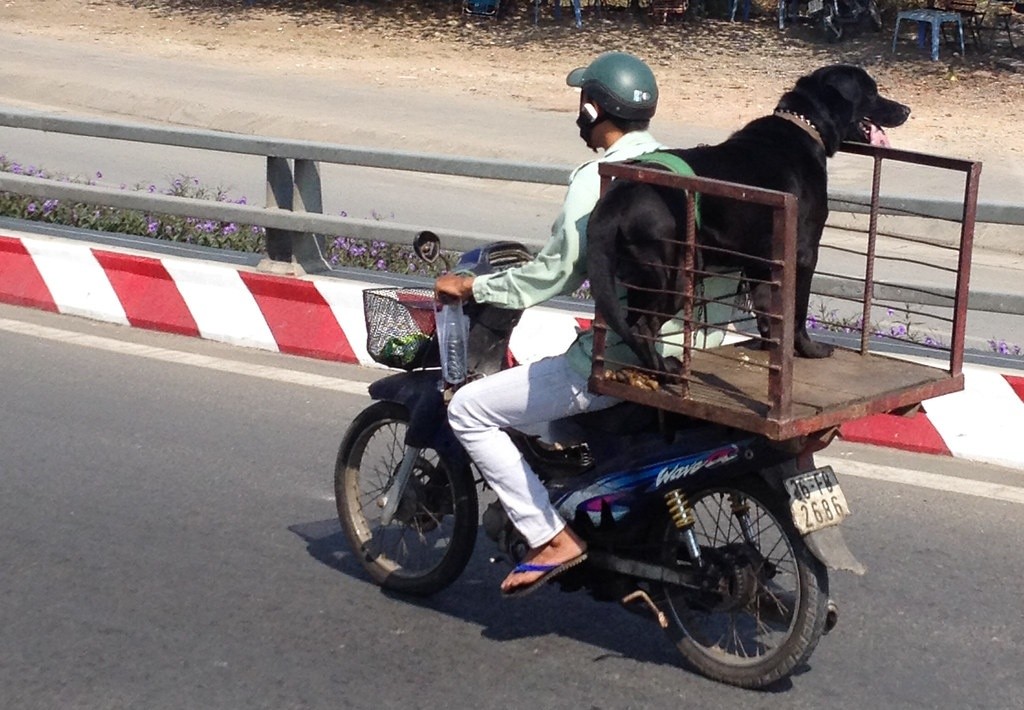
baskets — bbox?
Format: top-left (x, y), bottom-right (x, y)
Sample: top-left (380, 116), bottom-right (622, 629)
top-left (361, 286), bottom-right (441, 368)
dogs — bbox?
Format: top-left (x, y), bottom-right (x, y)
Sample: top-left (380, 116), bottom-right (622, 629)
top-left (585, 65), bottom-right (911, 384)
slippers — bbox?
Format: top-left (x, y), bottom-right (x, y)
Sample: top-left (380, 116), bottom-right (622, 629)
top-left (500, 550), bottom-right (587, 600)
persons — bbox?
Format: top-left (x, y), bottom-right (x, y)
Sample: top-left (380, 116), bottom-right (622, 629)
top-left (431, 51), bottom-right (742, 601)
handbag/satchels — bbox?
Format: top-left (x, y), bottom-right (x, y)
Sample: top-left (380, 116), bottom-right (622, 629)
top-left (433, 295), bottom-right (469, 384)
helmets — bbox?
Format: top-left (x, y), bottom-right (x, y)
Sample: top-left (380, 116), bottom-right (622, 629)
top-left (566, 52), bottom-right (658, 120)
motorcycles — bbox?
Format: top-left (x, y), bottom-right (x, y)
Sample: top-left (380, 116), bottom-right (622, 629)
top-left (329, 226), bottom-right (875, 686)
